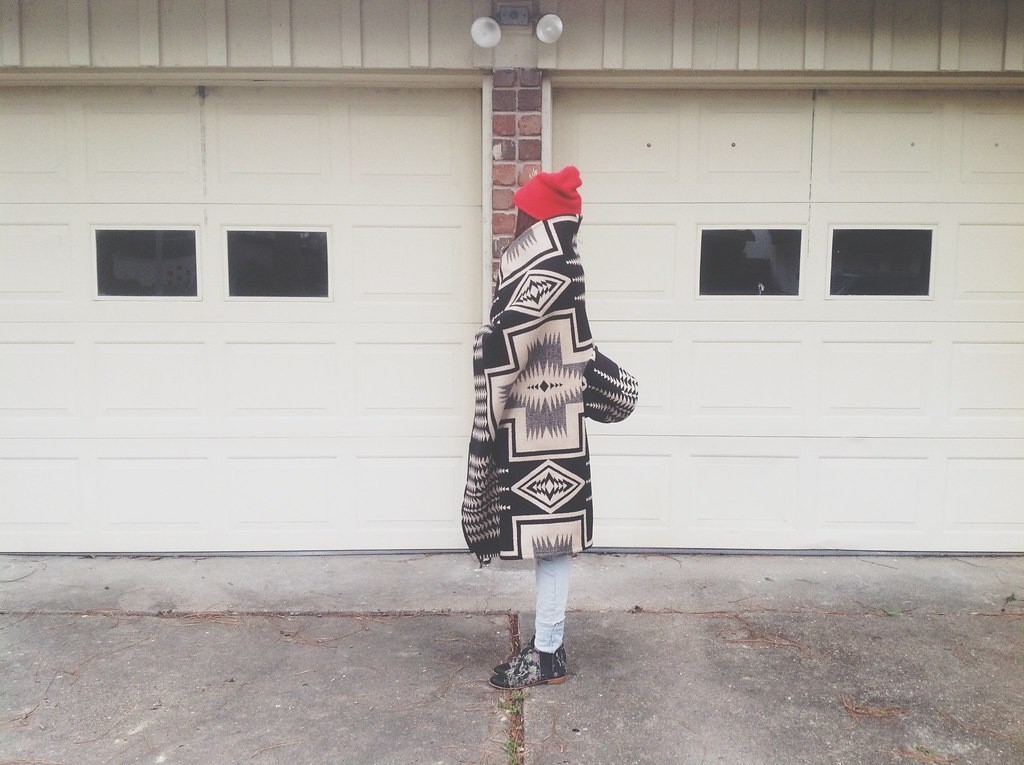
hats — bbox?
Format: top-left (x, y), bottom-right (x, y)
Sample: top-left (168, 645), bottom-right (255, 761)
top-left (512, 165), bottom-right (583, 220)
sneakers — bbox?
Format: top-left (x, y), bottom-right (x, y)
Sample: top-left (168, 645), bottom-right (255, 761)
top-left (493, 635), bottom-right (535, 672)
top-left (489, 645), bottom-right (566, 689)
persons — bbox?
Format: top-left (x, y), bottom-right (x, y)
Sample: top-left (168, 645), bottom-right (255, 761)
top-left (460, 165), bottom-right (640, 692)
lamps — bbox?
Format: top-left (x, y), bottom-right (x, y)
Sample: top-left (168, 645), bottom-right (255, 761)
top-left (531, 10), bottom-right (564, 45)
top-left (470, 13), bottom-right (506, 49)
